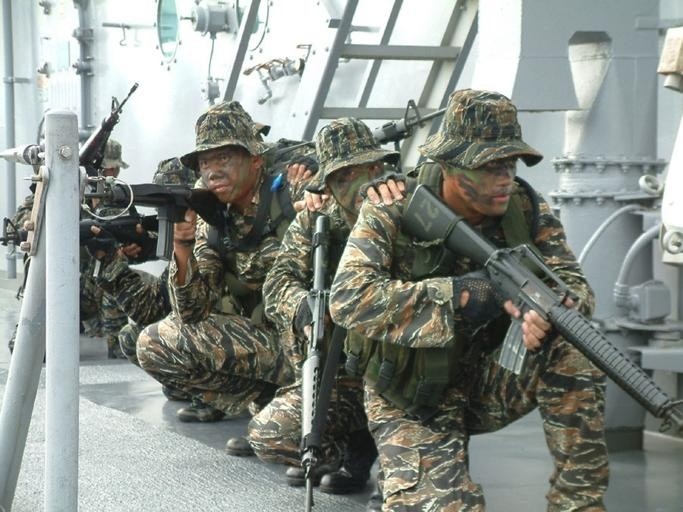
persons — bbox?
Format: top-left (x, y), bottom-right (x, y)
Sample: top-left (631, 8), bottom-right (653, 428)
top-left (328, 87), bottom-right (617, 511)
top-left (132, 100), bottom-right (318, 457)
top-left (248, 118), bottom-right (413, 495)
top-left (84, 153), bottom-right (204, 402)
top-left (4, 136), bottom-right (135, 363)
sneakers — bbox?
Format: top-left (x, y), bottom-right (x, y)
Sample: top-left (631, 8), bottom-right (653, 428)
top-left (286, 460), bottom-right (366, 493)
top-left (175, 396), bottom-right (227, 421)
top-left (226, 435), bottom-right (254, 456)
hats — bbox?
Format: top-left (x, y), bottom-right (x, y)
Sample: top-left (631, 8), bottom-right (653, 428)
top-left (304, 116), bottom-right (401, 193)
top-left (99, 138), bottom-right (129, 170)
top-left (418, 89), bottom-right (544, 169)
top-left (180, 100), bottom-right (277, 171)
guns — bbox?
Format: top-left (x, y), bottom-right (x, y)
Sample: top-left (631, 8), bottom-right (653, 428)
top-left (268, 100), bottom-right (447, 190)
top-left (83, 183), bottom-right (227, 259)
top-left (79, 82), bottom-right (138, 174)
top-left (0, 215), bottom-right (159, 246)
top-left (403, 184), bottom-right (683, 437)
top-left (296, 215), bottom-right (330, 512)
